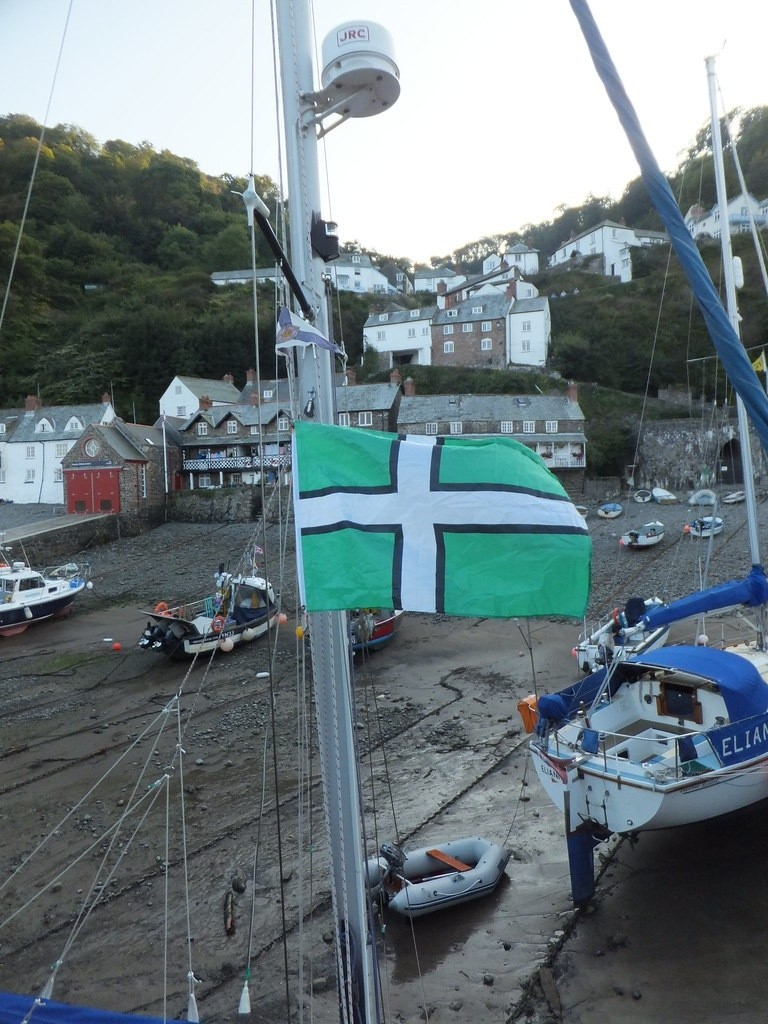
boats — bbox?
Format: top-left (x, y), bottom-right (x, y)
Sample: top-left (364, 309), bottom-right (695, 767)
top-left (596, 503), bottom-right (623, 520)
top-left (515, 56), bottom-right (768, 839)
top-left (688, 488), bottom-right (716, 507)
top-left (575, 505), bottom-right (589, 520)
top-left (356, 839), bottom-right (514, 920)
top-left (651, 486), bottom-right (679, 504)
top-left (293, 607), bottom-right (408, 657)
top-left (0, 551), bottom-right (92, 637)
top-left (722, 490), bottom-right (746, 504)
top-left (139, 572), bottom-right (285, 659)
top-left (619, 520), bottom-right (664, 551)
top-left (687, 516), bottom-right (724, 537)
top-left (634, 490), bottom-right (651, 504)
top-left (567, 595), bottom-right (676, 674)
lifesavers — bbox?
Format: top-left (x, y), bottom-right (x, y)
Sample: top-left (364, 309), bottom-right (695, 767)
top-left (209, 615), bottom-right (225, 633)
top-left (612, 607), bottom-right (623, 627)
top-left (153, 602), bottom-right (168, 614)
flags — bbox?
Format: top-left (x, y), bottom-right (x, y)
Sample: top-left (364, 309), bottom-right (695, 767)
top-left (254, 544), bottom-right (263, 555)
top-left (289, 418), bottom-right (595, 619)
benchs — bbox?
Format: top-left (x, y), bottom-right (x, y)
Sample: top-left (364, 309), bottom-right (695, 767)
top-left (598, 720), bottom-right (697, 764)
top-left (427, 850), bottom-right (472, 872)
